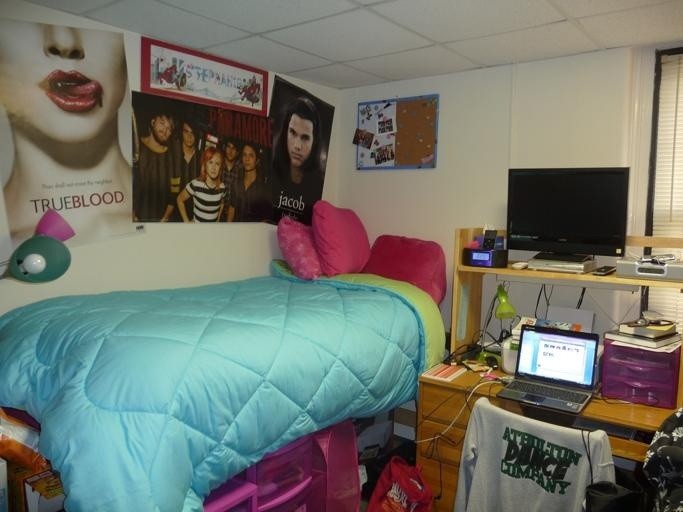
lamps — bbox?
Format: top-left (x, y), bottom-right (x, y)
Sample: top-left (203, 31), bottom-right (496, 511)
top-left (476, 284), bottom-right (516, 364)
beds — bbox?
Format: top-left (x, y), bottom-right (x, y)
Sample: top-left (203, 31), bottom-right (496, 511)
top-left (0, 260), bottom-right (446, 512)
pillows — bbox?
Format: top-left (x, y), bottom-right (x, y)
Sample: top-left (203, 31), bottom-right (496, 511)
top-left (312, 200), bottom-right (371, 277)
top-left (360, 235), bottom-right (447, 307)
top-left (278, 216), bottom-right (322, 280)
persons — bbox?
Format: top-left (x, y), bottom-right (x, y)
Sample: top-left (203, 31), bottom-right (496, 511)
top-left (219, 138), bottom-right (241, 221)
top-left (175, 147), bottom-right (227, 223)
top-left (0, 18), bottom-right (139, 267)
top-left (225, 141), bottom-right (267, 222)
top-left (131, 109), bottom-right (181, 222)
top-left (273, 99), bottom-right (323, 227)
top-left (174, 117), bottom-right (202, 224)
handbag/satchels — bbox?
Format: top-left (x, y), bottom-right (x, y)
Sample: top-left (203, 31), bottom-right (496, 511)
top-left (366, 455), bottom-right (436, 512)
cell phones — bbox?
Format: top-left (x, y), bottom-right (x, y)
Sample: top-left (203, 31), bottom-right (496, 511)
top-left (592, 266), bottom-right (616, 276)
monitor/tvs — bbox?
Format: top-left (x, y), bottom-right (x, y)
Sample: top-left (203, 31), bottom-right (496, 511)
top-left (508, 167), bottom-right (629, 263)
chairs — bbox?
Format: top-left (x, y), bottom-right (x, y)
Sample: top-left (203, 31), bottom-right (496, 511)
top-left (453, 397), bottom-right (616, 512)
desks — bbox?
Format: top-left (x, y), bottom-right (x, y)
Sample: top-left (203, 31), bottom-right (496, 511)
top-left (416, 227), bottom-right (683, 512)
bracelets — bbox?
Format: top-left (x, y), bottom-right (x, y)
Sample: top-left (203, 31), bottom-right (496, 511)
top-left (160, 217), bottom-right (167, 222)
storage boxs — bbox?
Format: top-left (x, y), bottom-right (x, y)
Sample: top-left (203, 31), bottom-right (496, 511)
top-left (601, 339), bottom-right (680, 410)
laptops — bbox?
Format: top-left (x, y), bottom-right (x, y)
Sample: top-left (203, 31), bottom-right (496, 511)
top-left (500, 326), bottom-right (600, 415)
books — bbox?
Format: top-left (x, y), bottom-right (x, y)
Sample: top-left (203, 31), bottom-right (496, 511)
top-left (603, 329), bottom-right (680, 349)
top-left (509, 315), bottom-right (582, 350)
top-left (618, 319), bottom-right (677, 339)
